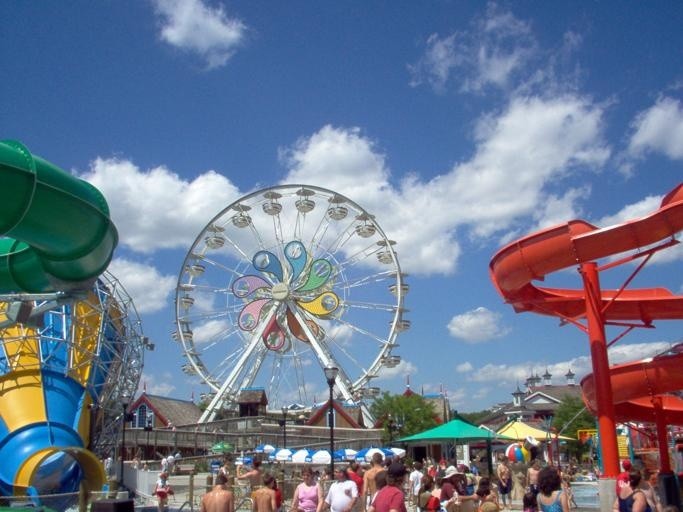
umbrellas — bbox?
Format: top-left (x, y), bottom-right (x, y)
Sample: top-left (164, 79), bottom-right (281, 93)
top-left (393, 418), bottom-right (512, 465)
top-left (495, 418), bottom-right (578, 443)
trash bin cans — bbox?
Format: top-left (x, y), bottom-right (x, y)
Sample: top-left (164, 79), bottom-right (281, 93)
top-left (104, 458), bottom-right (113, 479)
top-left (211, 461), bottom-right (222, 473)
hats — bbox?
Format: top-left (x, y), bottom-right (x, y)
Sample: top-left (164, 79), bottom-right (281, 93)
top-left (442, 466), bottom-right (464, 479)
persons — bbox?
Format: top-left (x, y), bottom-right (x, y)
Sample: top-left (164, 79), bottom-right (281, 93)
top-left (105, 448), bottom-right (682, 512)
